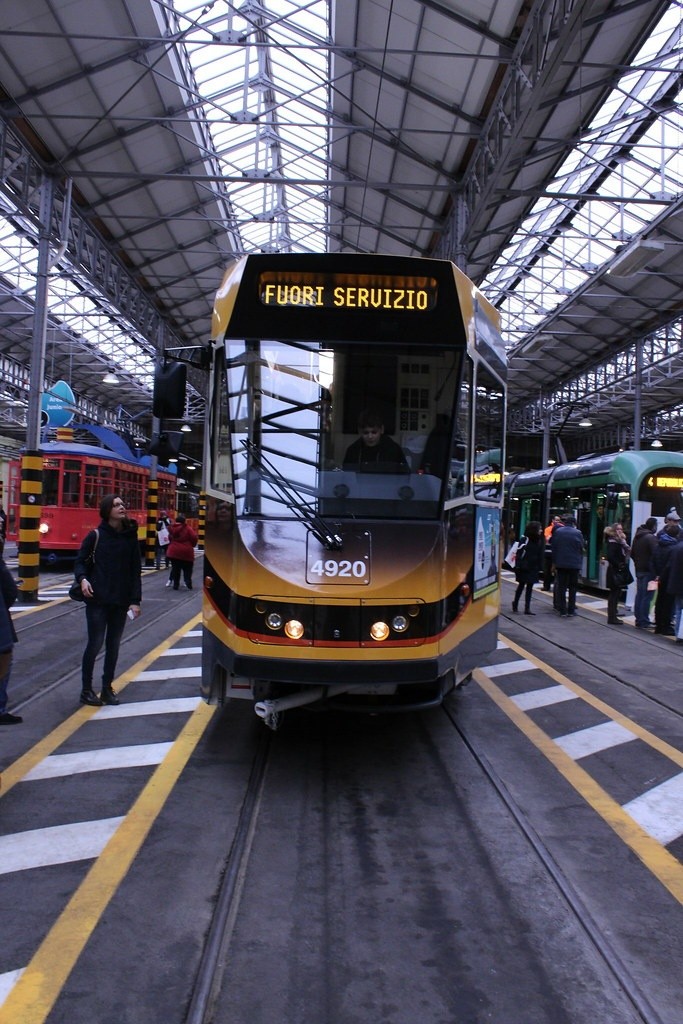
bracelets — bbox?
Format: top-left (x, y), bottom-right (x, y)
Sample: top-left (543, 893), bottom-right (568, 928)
top-left (80, 579), bottom-right (86, 582)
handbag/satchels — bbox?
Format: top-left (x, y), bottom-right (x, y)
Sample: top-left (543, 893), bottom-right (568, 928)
top-left (505, 542), bottom-right (526, 569)
top-left (157, 522), bottom-right (171, 546)
top-left (69, 580), bottom-right (87, 603)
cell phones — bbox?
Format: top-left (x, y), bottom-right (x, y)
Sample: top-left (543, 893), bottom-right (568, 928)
top-left (127, 609), bottom-right (134, 620)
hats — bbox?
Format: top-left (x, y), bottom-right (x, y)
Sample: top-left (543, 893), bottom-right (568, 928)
top-left (668, 513), bottom-right (681, 520)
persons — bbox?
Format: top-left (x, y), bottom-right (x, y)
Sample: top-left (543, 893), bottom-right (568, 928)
top-left (605, 523), bottom-right (634, 625)
top-left (0, 506), bottom-right (23, 725)
top-left (155, 510), bottom-right (172, 569)
top-left (630, 518), bottom-right (658, 628)
top-left (73, 494), bottom-right (142, 706)
top-left (654, 512), bottom-right (683, 646)
top-left (542, 513), bottom-right (579, 609)
top-left (342, 415), bottom-right (410, 474)
top-left (552, 517), bottom-right (584, 617)
top-left (163, 515), bottom-right (198, 590)
top-left (511, 521), bottom-right (543, 616)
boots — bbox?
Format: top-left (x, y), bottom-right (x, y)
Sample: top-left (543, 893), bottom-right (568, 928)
top-left (525, 604), bottom-right (536, 616)
top-left (511, 601), bottom-right (519, 613)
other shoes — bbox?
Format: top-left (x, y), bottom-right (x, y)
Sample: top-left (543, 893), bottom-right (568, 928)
top-left (663, 629), bottom-right (675, 635)
top-left (155, 567), bottom-right (161, 571)
top-left (174, 586), bottom-right (179, 590)
top-left (654, 627), bottom-right (662, 634)
top-left (643, 622), bottom-right (657, 629)
top-left (560, 612), bottom-right (569, 616)
top-left (568, 612), bottom-right (577, 616)
top-left (635, 623), bottom-right (642, 628)
top-left (188, 585), bottom-right (193, 590)
top-left (541, 586), bottom-right (553, 592)
top-left (1, 713), bottom-right (25, 726)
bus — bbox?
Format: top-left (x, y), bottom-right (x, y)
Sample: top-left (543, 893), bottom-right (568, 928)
top-left (5, 426), bottom-right (198, 573)
top-left (153, 253), bottom-right (507, 719)
top-left (502, 447), bottom-right (683, 590)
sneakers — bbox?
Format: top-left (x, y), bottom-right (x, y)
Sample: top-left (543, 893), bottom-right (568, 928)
top-left (166, 579), bottom-right (173, 586)
top-left (81, 691), bottom-right (104, 704)
top-left (609, 618), bottom-right (624, 625)
top-left (100, 686), bottom-right (120, 705)
